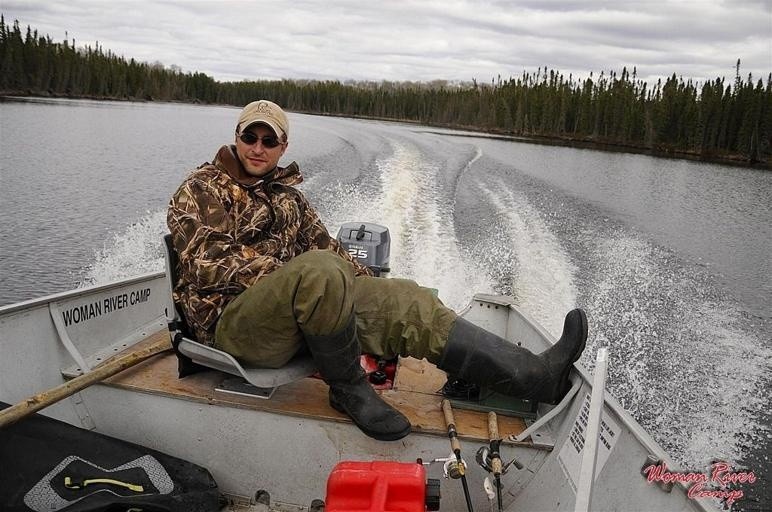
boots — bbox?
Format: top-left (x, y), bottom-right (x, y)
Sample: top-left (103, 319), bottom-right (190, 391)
top-left (435, 309), bottom-right (589, 404)
top-left (300, 305), bottom-right (411, 442)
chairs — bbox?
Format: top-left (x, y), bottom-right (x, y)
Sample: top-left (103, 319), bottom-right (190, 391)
top-left (161, 233), bottom-right (319, 388)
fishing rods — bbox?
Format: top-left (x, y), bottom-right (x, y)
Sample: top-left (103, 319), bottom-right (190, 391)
top-left (417, 399), bottom-right (474, 511)
top-left (475, 410), bottom-right (523, 511)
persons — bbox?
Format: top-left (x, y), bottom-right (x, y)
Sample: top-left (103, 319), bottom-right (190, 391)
top-left (164, 99), bottom-right (588, 441)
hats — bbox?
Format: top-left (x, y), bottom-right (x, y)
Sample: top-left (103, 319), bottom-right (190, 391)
top-left (238, 99), bottom-right (288, 138)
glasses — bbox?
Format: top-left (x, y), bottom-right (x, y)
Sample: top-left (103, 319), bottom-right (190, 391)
top-left (239, 128), bottom-right (281, 148)
